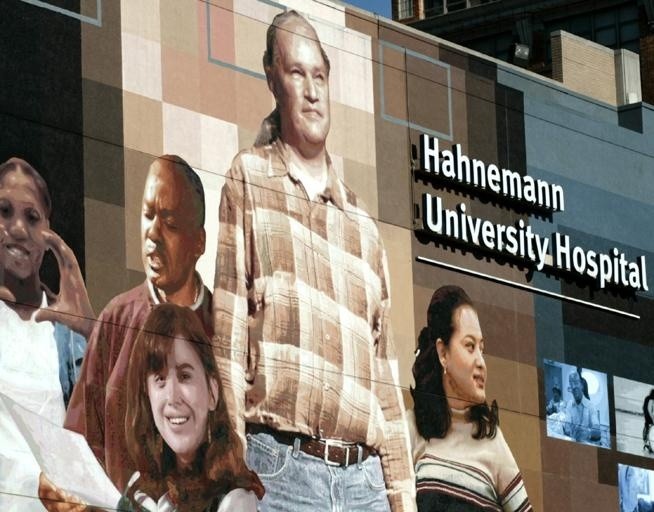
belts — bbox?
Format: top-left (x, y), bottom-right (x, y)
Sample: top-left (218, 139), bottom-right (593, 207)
top-left (248, 424), bottom-right (377, 467)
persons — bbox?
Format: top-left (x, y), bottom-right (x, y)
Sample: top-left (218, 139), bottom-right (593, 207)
top-left (619, 463), bottom-right (654, 512)
top-left (108, 303), bottom-right (269, 511)
top-left (207, 9), bottom-right (420, 512)
top-left (642, 388), bottom-right (654, 454)
top-left (404, 283), bottom-right (533, 509)
top-left (545, 384), bottom-right (565, 414)
top-left (0, 155), bottom-right (94, 509)
top-left (563, 372), bottom-right (601, 442)
top-left (34, 152), bottom-right (212, 509)
top-left (576, 366), bottom-right (589, 398)
